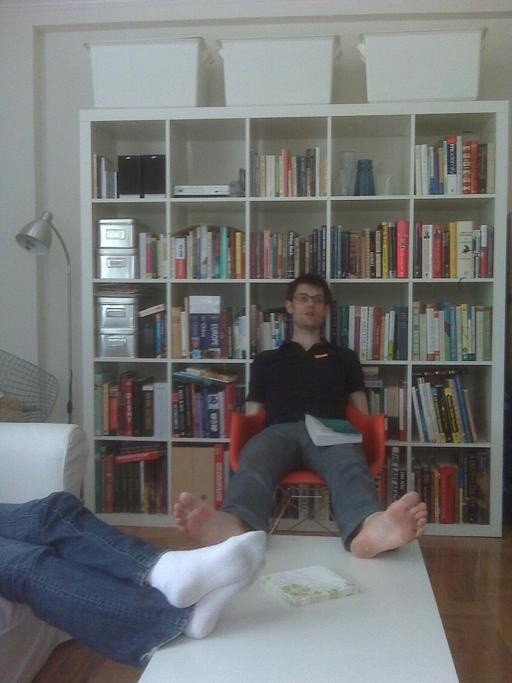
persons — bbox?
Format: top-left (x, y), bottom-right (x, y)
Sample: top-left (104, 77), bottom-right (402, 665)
top-left (171, 272), bottom-right (430, 559)
top-left (0, 491), bottom-right (268, 670)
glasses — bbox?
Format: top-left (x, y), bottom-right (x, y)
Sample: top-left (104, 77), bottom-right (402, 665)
top-left (293, 293), bottom-right (325, 304)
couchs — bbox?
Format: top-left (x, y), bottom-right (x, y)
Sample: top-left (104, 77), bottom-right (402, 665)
top-left (1, 417), bottom-right (86, 682)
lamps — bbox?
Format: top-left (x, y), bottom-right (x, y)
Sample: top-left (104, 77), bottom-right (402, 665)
top-left (13, 210), bottom-right (76, 423)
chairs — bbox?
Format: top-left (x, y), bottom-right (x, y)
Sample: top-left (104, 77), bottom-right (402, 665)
top-left (227, 402), bottom-right (386, 534)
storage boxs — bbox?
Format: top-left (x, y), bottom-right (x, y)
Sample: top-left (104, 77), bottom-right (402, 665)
top-left (98, 326), bottom-right (141, 358)
top-left (96, 294), bottom-right (143, 329)
top-left (213, 33), bottom-right (341, 107)
top-left (96, 248), bottom-right (140, 279)
top-left (356, 24), bottom-right (490, 102)
top-left (81, 33), bottom-right (212, 108)
top-left (97, 217), bottom-right (141, 248)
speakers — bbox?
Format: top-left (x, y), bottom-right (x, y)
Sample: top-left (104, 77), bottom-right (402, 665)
top-left (117, 155), bottom-right (141, 199)
top-left (142, 154), bottom-right (166, 198)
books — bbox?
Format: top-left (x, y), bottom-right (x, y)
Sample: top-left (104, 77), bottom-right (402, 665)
top-left (250, 147), bottom-right (325, 196)
top-left (414, 135), bottom-right (495, 193)
top-left (330, 220), bottom-right (410, 278)
top-left (251, 305), bottom-right (293, 359)
top-left (139, 224), bottom-right (246, 279)
top-left (171, 295), bottom-right (246, 358)
top-left (413, 220), bottom-right (494, 525)
top-left (172, 366), bottom-right (244, 439)
top-left (258, 564), bottom-right (357, 607)
top-left (93, 153), bottom-right (117, 198)
top-left (331, 298), bottom-right (408, 361)
top-left (249, 224), bottom-right (327, 279)
top-left (95, 371), bottom-right (168, 436)
top-left (95, 441), bottom-right (168, 514)
top-left (360, 366), bottom-right (407, 442)
top-left (210, 444), bottom-right (407, 518)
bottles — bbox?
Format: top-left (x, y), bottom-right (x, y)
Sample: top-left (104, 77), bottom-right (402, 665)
top-left (335, 151), bottom-right (393, 197)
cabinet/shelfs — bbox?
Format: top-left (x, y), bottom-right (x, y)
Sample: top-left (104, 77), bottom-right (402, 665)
top-left (78, 96), bottom-right (512, 539)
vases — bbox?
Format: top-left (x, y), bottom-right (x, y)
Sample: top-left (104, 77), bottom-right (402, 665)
top-left (330, 149), bottom-right (357, 197)
top-left (352, 158), bottom-right (376, 195)
